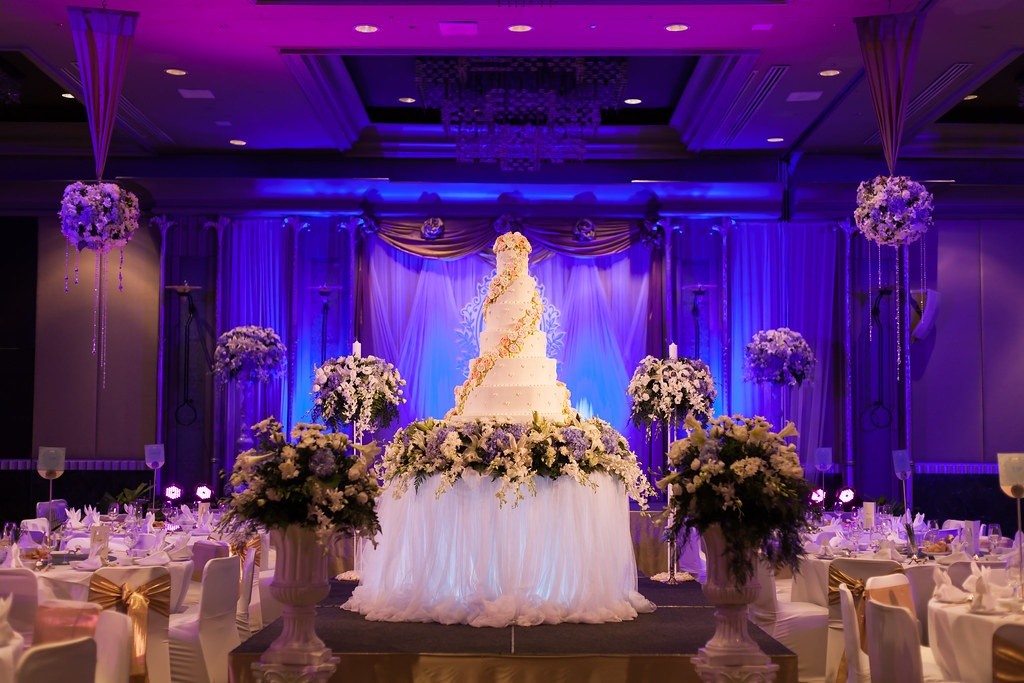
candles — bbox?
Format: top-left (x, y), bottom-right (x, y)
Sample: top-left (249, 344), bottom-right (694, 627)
top-left (352, 338), bottom-right (362, 359)
top-left (668, 342), bottom-right (678, 361)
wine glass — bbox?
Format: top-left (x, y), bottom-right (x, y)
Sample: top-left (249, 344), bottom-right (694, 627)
top-left (40, 496), bottom-right (258, 568)
top-left (773, 500), bottom-right (1024, 619)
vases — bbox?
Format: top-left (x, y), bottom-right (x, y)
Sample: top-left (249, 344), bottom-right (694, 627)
top-left (696, 522), bottom-right (773, 669)
top-left (259, 523), bottom-right (337, 666)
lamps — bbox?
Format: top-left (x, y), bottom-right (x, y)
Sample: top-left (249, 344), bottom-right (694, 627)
top-left (413, 55), bottom-right (629, 175)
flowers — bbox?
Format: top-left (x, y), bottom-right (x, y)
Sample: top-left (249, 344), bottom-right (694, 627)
top-left (742, 328), bottom-right (818, 390)
top-left (205, 325), bottom-right (289, 398)
top-left (645, 410), bottom-right (823, 599)
top-left (492, 214), bottom-right (524, 235)
top-left (421, 217), bottom-right (445, 240)
top-left (624, 355), bottom-right (718, 447)
top-left (492, 231), bottom-right (533, 255)
top-left (56, 181), bottom-right (142, 256)
top-left (444, 250), bottom-right (544, 420)
top-left (355, 213), bottom-right (381, 239)
top-left (572, 217), bottom-right (598, 242)
top-left (299, 352), bottom-right (408, 440)
top-left (639, 215), bottom-right (668, 252)
top-left (379, 414), bottom-right (660, 519)
top-left (853, 175), bottom-right (934, 249)
top-left (208, 413), bottom-right (383, 556)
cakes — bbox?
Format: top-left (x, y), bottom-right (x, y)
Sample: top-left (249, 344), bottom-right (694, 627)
top-left (444, 231), bottom-right (572, 429)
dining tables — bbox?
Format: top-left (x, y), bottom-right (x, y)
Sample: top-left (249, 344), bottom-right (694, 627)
top-left (790, 551), bottom-right (947, 630)
top-left (926, 594), bottom-right (1024, 683)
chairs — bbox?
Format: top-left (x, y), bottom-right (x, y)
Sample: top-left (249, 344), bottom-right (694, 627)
top-left (823, 519), bottom-right (1024, 683)
top-left (0, 498), bottom-right (270, 683)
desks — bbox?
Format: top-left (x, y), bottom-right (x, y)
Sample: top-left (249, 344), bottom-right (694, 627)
top-left (20, 550), bottom-right (195, 617)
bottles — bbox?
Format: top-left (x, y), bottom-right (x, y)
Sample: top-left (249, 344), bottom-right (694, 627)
top-left (3, 522), bottom-right (17, 547)
